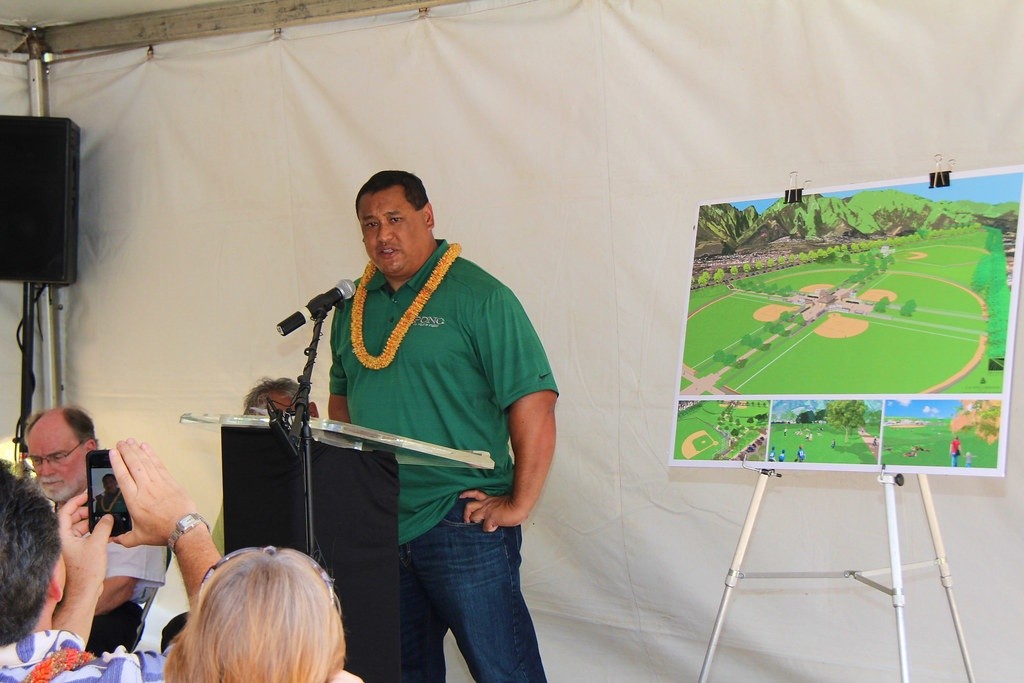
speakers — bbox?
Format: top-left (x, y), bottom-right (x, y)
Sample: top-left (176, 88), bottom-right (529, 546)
top-left (0, 115), bottom-right (79, 284)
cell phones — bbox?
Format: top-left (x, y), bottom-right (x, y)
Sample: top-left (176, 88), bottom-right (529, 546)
top-left (85, 449), bottom-right (133, 537)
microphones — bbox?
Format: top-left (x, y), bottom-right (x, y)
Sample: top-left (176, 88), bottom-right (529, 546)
top-left (276, 279), bottom-right (357, 337)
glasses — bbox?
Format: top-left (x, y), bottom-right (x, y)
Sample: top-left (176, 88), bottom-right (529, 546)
top-left (198, 546), bottom-right (336, 606)
top-left (23, 442), bottom-right (81, 470)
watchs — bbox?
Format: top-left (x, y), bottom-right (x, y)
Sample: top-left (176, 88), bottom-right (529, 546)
top-left (167, 512), bottom-right (211, 556)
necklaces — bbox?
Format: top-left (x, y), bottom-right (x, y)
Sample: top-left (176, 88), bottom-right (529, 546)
top-left (350, 243), bottom-right (462, 369)
top-left (100, 488), bottom-right (122, 511)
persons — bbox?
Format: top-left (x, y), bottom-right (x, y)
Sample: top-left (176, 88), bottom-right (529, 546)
top-left (160, 376), bottom-right (320, 653)
top-left (327, 170), bottom-right (560, 683)
top-left (95, 473), bottom-right (126, 512)
top-left (1, 407), bottom-right (366, 682)
